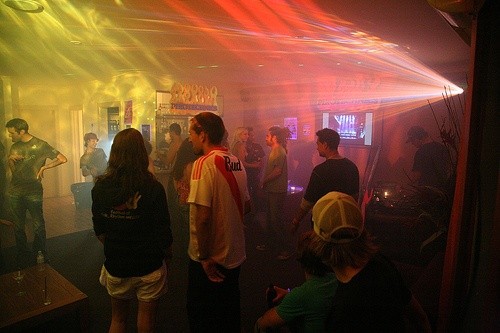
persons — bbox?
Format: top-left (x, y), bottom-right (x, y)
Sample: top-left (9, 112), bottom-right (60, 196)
top-left (288, 128), bottom-right (360, 237)
top-left (256, 191), bottom-right (436, 333)
top-left (92, 129), bottom-right (172, 333)
top-left (7, 117), bottom-right (66, 270)
top-left (165, 112), bottom-right (293, 333)
top-left (80, 133), bottom-right (110, 181)
top-left (406, 127), bottom-right (454, 186)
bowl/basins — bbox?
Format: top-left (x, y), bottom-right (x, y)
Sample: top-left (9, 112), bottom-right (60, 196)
top-left (3, 272), bottom-right (42, 290)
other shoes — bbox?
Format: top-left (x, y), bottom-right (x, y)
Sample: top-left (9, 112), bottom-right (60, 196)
top-left (41, 249), bottom-right (49, 263)
top-left (15, 251), bottom-right (27, 270)
top-left (277, 250), bottom-right (294, 260)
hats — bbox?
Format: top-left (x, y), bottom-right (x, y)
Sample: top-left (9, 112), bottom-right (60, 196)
top-left (84, 133), bottom-right (99, 142)
top-left (405, 127), bottom-right (428, 143)
top-left (312, 191), bottom-right (365, 243)
top-left (191, 112), bottom-right (225, 145)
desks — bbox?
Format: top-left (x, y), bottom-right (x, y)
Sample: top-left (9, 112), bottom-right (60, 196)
top-left (0, 262), bottom-right (90, 333)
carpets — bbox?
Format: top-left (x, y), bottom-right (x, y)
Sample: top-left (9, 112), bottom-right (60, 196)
top-left (0, 229), bottom-right (308, 333)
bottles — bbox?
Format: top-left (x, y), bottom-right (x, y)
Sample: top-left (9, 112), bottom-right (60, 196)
top-left (36, 250), bottom-right (45, 272)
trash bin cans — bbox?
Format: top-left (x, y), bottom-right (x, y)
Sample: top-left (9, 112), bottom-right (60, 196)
top-left (72, 181), bottom-right (93, 209)
